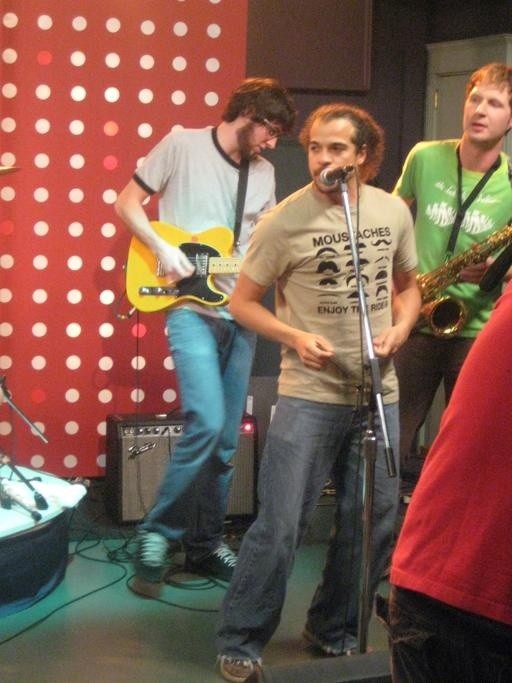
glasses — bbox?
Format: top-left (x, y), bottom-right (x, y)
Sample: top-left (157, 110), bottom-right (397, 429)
top-left (251, 111), bottom-right (284, 140)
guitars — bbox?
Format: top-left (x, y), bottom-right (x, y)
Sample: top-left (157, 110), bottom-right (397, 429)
top-left (125, 221), bottom-right (246, 313)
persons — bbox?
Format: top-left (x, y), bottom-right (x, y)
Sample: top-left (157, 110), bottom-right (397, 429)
top-left (208, 101), bottom-right (427, 683)
top-left (384, 270), bottom-right (511, 680)
top-left (384, 63), bottom-right (511, 491)
top-left (112, 73), bottom-right (302, 585)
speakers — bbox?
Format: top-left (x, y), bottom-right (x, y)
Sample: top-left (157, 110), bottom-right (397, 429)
top-left (107, 411), bottom-right (259, 526)
top-left (242, 649), bottom-right (393, 683)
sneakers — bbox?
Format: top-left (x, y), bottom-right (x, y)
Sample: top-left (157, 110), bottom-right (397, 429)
top-left (138, 529), bottom-right (171, 582)
top-left (299, 625), bottom-right (372, 661)
top-left (216, 650), bottom-right (264, 683)
top-left (186, 543), bottom-right (237, 579)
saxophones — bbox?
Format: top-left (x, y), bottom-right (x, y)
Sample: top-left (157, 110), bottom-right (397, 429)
top-left (413, 223), bottom-right (512, 339)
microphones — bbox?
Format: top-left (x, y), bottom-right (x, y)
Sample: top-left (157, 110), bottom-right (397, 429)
top-left (319, 164), bottom-right (354, 187)
